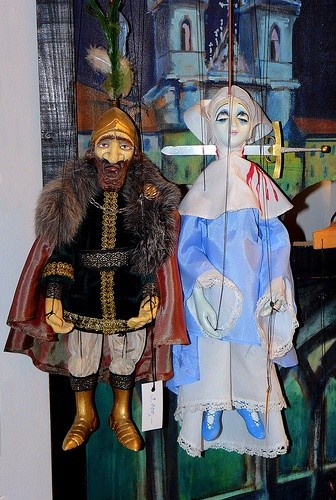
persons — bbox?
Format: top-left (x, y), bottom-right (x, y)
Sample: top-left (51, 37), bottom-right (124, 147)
top-left (1, 105), bottom-right (191, 452)
top-left (163, 85), bottom-right (302, 459)
